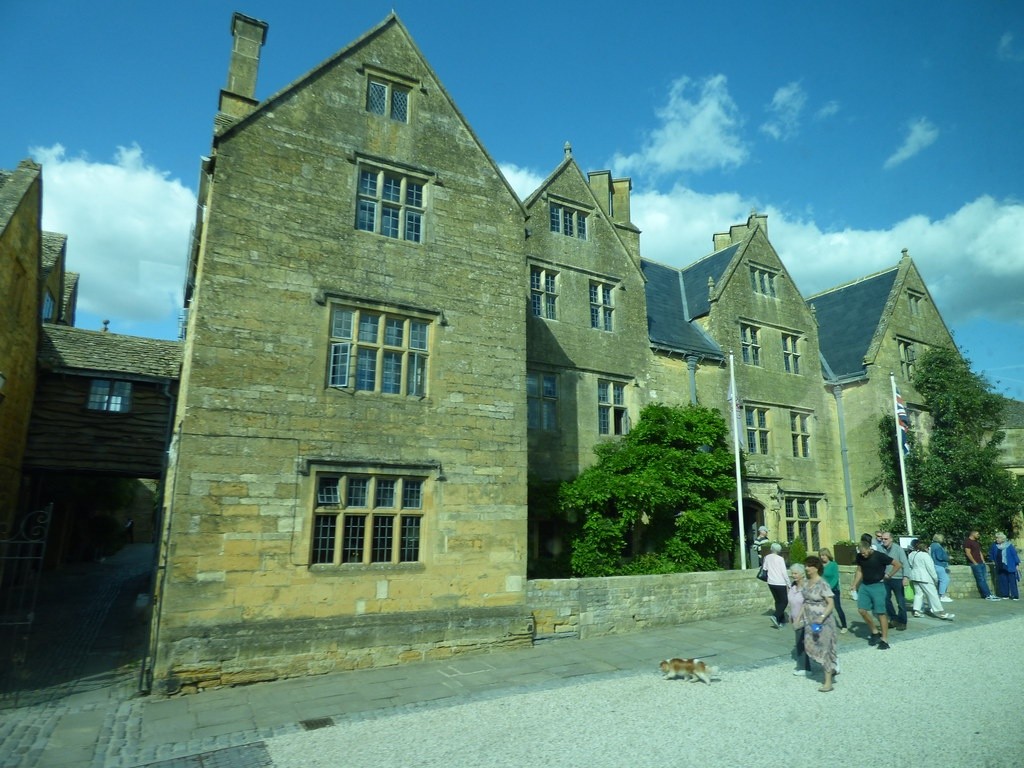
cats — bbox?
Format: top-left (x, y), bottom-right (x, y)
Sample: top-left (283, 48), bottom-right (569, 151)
top-left (659, 659), bottom-right (720, 686)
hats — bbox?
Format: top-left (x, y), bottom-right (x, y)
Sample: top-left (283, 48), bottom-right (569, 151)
top-left (758, 526), bottom-right (768, 532)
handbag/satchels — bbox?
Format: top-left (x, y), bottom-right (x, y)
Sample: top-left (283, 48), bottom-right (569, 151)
top-left (1017, 570), bottom-right (1022, 582)
top-left (904, 579), bottom-right (914, 603)
top-left (757, 568), bottom-right (768, 581)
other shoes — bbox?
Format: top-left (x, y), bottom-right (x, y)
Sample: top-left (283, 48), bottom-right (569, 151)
top-left (835, 659), bottom-right (841, 674)
top-left (770, 615), bottom-right (779, 629)
top-left (793, 669), bottom-right (813, 676)
top-left (985, 595), bottom-right (1001, 601)
top-left (940, 596), bottom-right (953, 602)
top-left (888, 620), bottom-right (898, 628)
top-left (876, 641), bottom-right (890, 650)
top-left (914, 611), bottom-right (925, 617)
top-left (896, 623), bottom-right (907, 630)
top-left (1002, 596), bottom-right (1010, 600)
top-left (819, 685), bottom-right (835, 692)
top-left (868, 631), bottom-right (882, 646)
top-left (840, 628), bottom-right (848, 633)
top-left (1013, 596), bottom-right (1019, 600)
top-left (940, 613), bottom-right (955, 619)
top-left (778, 622), bottom-right (783, 627)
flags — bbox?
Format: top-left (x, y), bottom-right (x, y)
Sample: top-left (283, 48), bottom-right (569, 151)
top-left (895, 386), bottom-right (910, 454)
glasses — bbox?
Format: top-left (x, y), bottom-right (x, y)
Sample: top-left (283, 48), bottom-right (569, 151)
top-left (879, 537), bottom-right (889, 540)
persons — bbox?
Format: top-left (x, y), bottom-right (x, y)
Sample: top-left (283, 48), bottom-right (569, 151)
top-left (753, 525), bottom-right (1022, 693)
